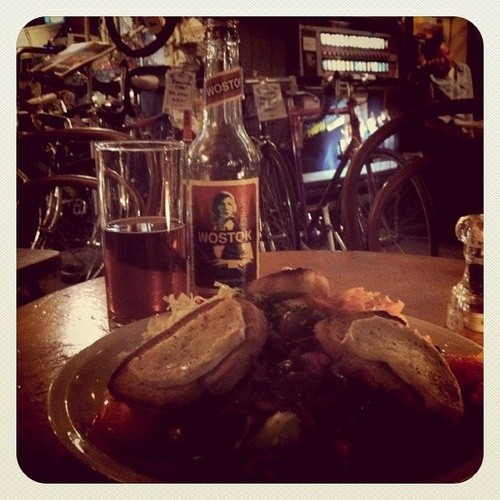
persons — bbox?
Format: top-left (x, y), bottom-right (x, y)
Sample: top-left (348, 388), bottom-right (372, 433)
top-left (406, 31), bottom-right (477, 243)
top-left (195, 191), bottom-right (255, 290)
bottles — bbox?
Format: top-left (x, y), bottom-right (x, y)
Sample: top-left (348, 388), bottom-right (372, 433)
top-left (183, 18), bottom-right (263, 304)
top-left (448, 215), bottom-right (484, 333)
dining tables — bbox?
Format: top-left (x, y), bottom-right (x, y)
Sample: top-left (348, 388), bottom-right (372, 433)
top-left (19, 247), bottom-right (484, 484)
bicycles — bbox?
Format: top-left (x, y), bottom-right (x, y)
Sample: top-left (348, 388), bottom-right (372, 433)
top-left (16, 17), bottom-right (483, 303)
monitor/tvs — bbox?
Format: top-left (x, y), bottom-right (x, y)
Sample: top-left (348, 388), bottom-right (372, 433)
top-left (282, 76), bottom-right (409, 199)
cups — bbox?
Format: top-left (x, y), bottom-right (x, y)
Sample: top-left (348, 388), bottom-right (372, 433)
top-left (94, 140), bottom-right (194, 329)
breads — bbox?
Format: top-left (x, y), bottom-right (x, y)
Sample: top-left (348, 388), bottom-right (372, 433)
top-left (108, 293), bottom-right (271, 410)
top-left (242, 267), bottom-right (330, 310)
top-left (303, 310), bottom-right (466, 421)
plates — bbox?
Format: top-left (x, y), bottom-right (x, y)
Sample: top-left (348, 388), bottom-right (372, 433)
top-left (46, 309), bottom-right (484, 483)
